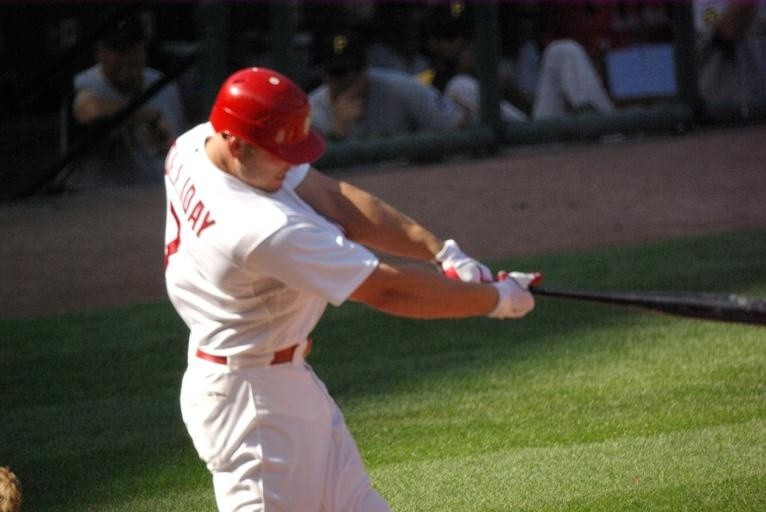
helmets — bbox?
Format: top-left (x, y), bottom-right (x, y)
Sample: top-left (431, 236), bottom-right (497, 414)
top-left (209, 66), bottom-right (327, 165)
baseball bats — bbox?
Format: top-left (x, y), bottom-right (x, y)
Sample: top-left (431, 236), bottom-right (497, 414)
top-left (529, 285), bottom-right (765, 324)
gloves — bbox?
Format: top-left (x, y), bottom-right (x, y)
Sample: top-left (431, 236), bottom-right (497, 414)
top-left (430, 239), bottom-right (493, 284)
top-left (487, 271), bottom-right (544, 320)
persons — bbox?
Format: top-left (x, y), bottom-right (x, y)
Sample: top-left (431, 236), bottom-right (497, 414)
top-left (163, 67), bottom-right (542, 512)
top-left (72, 0), bottom-right (766, 168)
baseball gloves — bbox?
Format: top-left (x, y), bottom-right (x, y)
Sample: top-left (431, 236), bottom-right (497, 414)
top-left (0, 466), bottom-right (22, 512)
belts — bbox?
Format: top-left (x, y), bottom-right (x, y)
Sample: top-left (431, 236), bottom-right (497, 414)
top-left (195, 338), bottom-right (313, 366)
